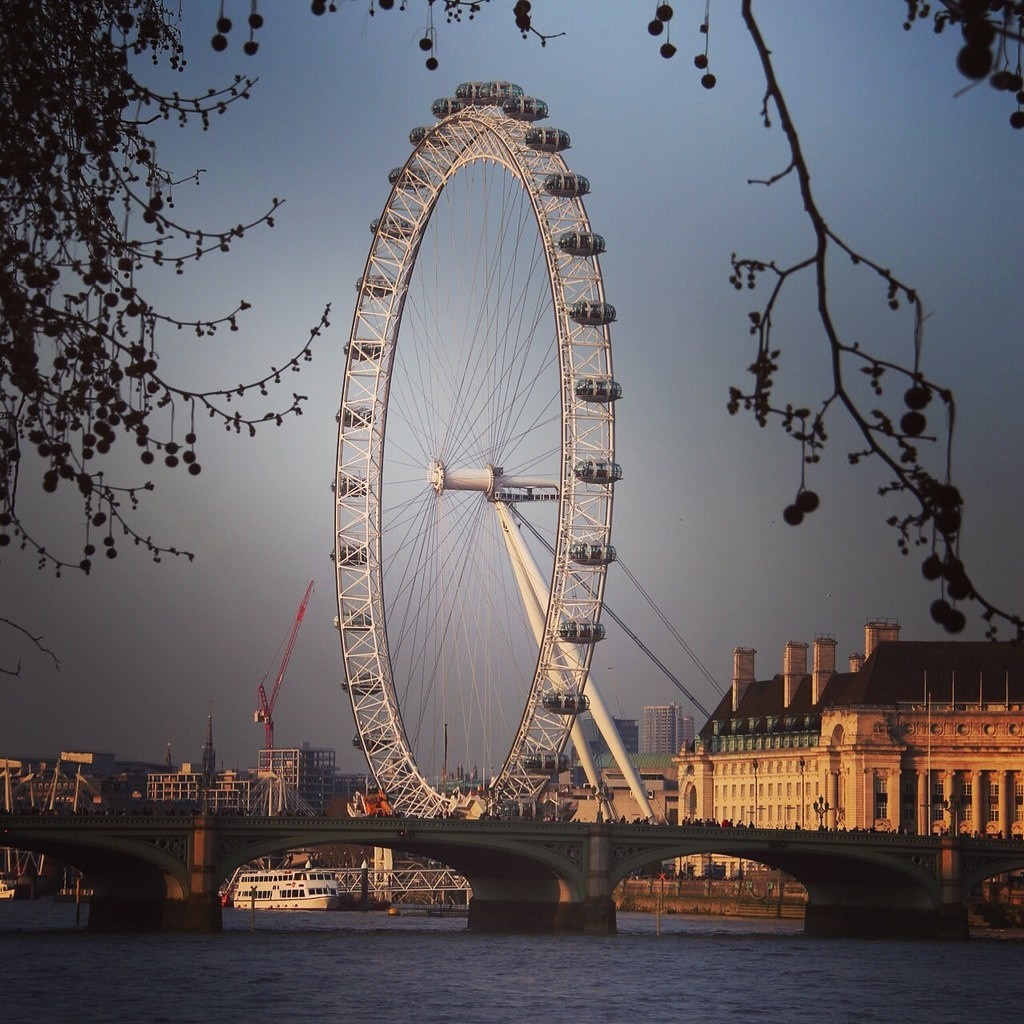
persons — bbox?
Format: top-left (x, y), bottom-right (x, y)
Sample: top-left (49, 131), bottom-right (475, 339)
top-left (1, 800), bottom-right (1024, 844)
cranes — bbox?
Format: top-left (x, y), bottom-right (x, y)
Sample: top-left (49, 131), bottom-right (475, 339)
top-left (251, 576), bottom-right (317, 770)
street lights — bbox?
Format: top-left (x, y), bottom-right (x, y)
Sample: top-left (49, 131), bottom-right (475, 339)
top-left (591, 779), bottom-right (611, 823)
top-left (753, 760), bottom-right (759, 828)
top-left (943, 795), bottom-right (960, 836)
top-left (479, 784), bottom-right (495, 820)
top-left (813, 795), bottom-right (829, 831)
top-left (799, 756), bottom-right (805, 828)
top-left (197, 770), bottom-right (216, 815)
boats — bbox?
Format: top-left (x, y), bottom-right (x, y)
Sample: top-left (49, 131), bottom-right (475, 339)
top-left (232, 867), bottom-right (341, 912)
